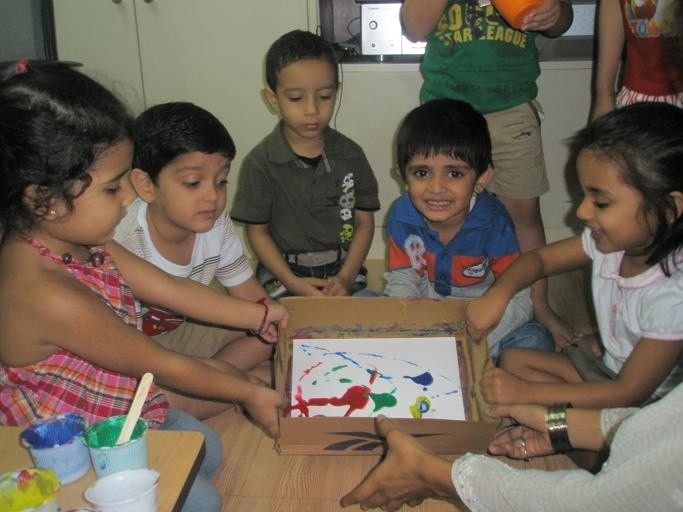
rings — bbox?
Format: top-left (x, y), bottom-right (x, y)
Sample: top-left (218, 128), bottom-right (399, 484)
top-left (522, 448), bottom-right (529, 458)
top-left (519, 437), bottom-right (525, 447)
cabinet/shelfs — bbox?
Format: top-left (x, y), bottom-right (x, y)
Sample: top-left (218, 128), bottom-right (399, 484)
top-left (38, 0), bottom-right (323, 258)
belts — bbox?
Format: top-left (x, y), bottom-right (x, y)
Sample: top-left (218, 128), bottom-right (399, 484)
top-left (281, 247), bottom-right (345, 267)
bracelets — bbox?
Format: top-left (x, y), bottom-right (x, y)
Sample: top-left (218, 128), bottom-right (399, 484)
top-left (248, 297), bottom-right (269, 335)
top-left (545, 406), bottom-right (574, 454)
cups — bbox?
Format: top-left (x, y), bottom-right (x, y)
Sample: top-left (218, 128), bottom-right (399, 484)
top-left (82, 414), bottom-right (147, 478)
top-left (0, 468), bottom-right (60, 512)
top-left (84, 468), bottom-right (160, 510)
top-left (19, 413), bottom-right (89, 484)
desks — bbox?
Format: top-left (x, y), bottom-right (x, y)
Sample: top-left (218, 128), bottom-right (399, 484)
top-left (0, 425), bottom-right (207, 512)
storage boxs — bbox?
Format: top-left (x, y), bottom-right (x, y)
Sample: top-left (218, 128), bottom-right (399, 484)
top-left (269, 294), bottom-right (504, 457)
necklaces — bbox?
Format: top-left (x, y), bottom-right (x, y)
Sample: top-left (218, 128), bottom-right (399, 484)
top-left (146, 207), bottom-right (192, 244)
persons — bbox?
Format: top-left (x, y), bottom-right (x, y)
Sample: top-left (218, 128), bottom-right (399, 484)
top-left (589, 1), bottom-right (682, 125)
top-left (354, 98), bottom-right (556, 367)
top-left (112, 100), bottom-right (276, 420)
top-left (400, 1), bottom-right (582, 349)
top-left (462, 100), bottom-right (683, 476)
top-left (0, 58), bottom-right (289, 511)
top-left (341, 378), bottom-right (683, 510)
top-left (226, 29), bottom-right (381, 296)
